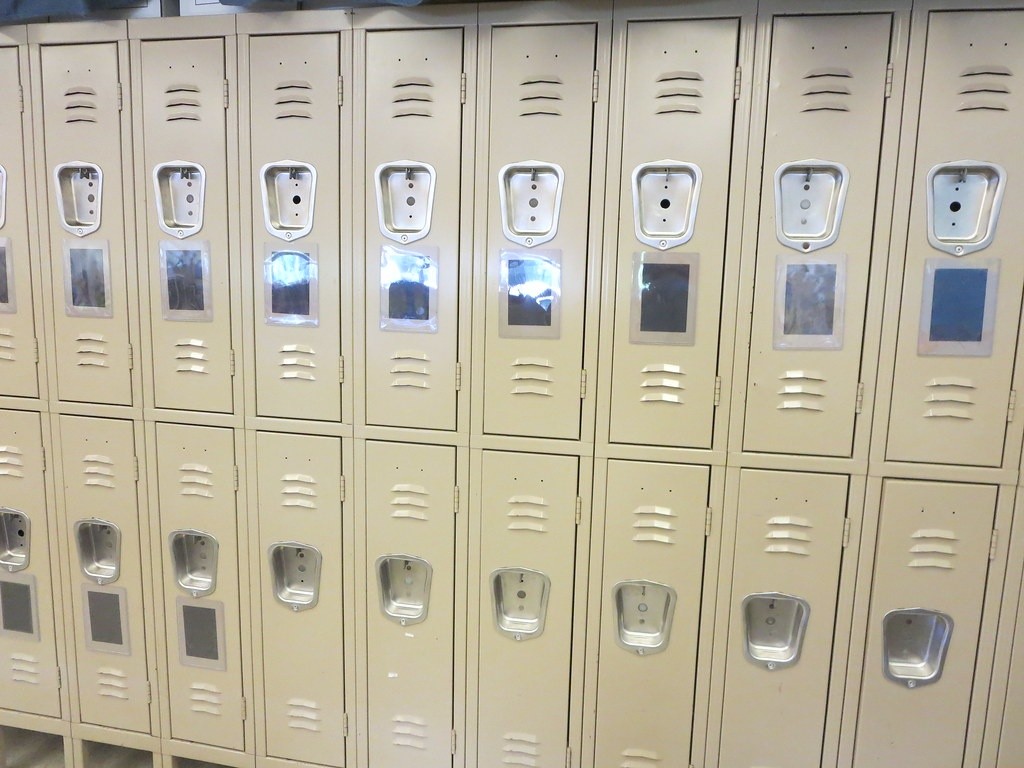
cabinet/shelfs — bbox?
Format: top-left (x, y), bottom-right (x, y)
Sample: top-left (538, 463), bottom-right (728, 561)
top-left (27, 19), bottom-right (162, 768)
top-left (579, 0), bottom-right (758, 768)
top-left (237, 7), bottom-right (369, 768)
top-left (127, 13), bottom-right (256, 768)
top-left (465, 0), bottom-right (612, 768)
top-left (836, 0), bottom-right (1024, 768)
top-left (0, 24), bottom-right (73, 768)
top-left (353, 2), bottom-right (479, 768)
top-left (704, 0), bottom-right (912, 768)
